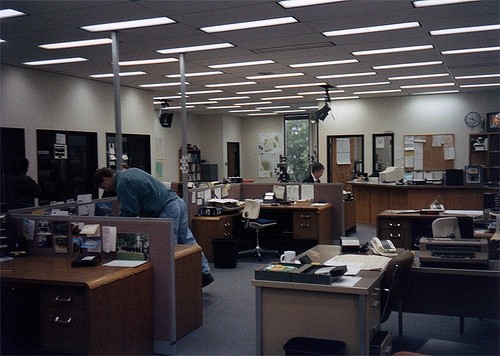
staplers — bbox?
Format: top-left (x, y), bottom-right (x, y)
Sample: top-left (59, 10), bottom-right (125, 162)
top-left (360, 241), bottom-right (369, 252)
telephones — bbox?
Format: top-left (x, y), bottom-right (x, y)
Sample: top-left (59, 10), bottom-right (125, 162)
top-left (371, 237), bottom-right (396, 254)
top-left (430, 202), bottom-right (446, 212)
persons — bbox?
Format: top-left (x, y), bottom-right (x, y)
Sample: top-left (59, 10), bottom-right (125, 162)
top-left (6, 157), bottom-right (42, 254)
top-left (303, 163), bottom-right (326, 183)
top-left (490, 115), bottom-right (500, 128)
top-left (95, 167), bottom-right (214, 288)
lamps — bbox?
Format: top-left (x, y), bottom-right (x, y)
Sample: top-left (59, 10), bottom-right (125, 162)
top-left (158, 104), bottom-right (174, 128)
top-left (309, 85), bottom-right (332, 123)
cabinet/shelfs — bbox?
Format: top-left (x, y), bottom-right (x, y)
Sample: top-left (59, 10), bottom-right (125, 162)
top-left (178, 149), bottom-right (202, 183)
top-left (469, 131), bottom-right (500, 183)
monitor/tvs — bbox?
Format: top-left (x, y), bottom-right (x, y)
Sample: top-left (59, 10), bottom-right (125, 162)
top-left (379, 166), bottom-right (404, 183)
top-left (484, 111), bottom-right (500, 134)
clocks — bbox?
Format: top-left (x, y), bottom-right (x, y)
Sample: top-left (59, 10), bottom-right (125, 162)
top-left (465, 111), bottom-right (482, 127)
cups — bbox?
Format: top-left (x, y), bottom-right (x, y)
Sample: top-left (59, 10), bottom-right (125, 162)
top-left (280, 251), bottom-right (295, 264)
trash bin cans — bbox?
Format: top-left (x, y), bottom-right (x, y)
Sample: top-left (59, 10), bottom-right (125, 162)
top-left (211, 237), bottom-right (240, 270)
top-left (284, 337), bottom-right (346, 356)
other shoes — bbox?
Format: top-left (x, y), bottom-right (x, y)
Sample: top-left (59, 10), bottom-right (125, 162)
top-left (202, 273), bottom-right (214, 287)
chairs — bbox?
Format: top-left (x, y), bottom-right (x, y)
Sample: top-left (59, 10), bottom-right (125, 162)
top-left (381, 250), bottom-right (413, 345)
top-left (238, 199), bottom-right (279, 259)
top-left (432, 217), bottom-right (462, 240)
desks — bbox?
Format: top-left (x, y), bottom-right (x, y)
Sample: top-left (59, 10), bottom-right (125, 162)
top-left (191, 196), bottom-right (355, 259)
top-left (252, 210), bottom-right (500, 356)
top-left (0, 243), bottom-right (202, 356)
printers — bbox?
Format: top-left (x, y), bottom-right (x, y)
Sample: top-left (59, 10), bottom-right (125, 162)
top-left (419, 237), bottom-right (488, 269)
